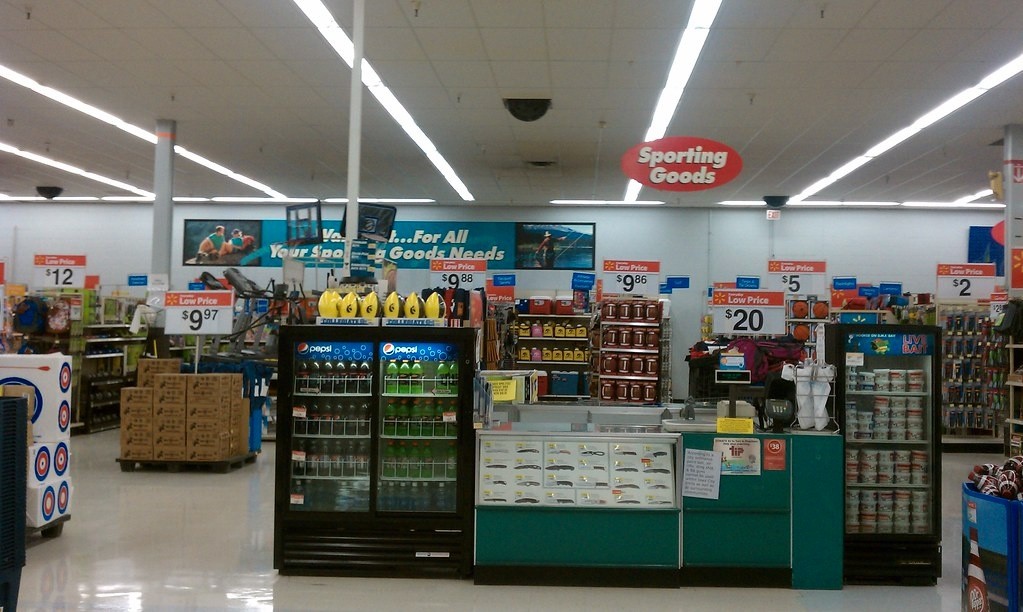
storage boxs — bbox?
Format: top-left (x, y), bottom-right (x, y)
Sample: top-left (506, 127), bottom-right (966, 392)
top-left (0, 353), bottom-right (72, 529)
top-left (120, 359), bottom-right (250, 461)
top-left (536, 370), bottom-right (548, 395)
top-left (551, 371), bottom-right (578, 395)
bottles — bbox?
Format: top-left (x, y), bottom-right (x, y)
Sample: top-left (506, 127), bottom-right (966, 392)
top-left (967, 456), bottom-right (1023, 502)
top-left (511, 320), bottom-right (587, 338)
top-left (966, 527), bottom-right (987, 612)
top-left (290, 360), bottom-right (458, 512)
top-left (518, 347), bottom-right (589, 361)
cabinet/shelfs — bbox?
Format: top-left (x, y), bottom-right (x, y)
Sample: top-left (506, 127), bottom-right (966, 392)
top-left (516, 314), bottom-right (592, 398)
top-left (785, 299), bottom-right (831, 364)
top-left (83, 324), bottom-right (147, 434)
top-left (599, 297), bottom-right (659, 405)
top-left (1004, 335), bottom-right (1023, 458)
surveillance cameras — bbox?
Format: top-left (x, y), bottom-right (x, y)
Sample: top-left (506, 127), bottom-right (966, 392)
top-left (37, 186), bottom-right (62, 199)
top-left (762, 196), bottom-right (790, 207)
top-left (503, 98), bottom-right (551, 122)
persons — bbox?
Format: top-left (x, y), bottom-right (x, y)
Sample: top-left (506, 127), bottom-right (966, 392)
top-left (197, 226), bottom-right (254, 264)
top-left (536, 231), bottom-right (566, 263)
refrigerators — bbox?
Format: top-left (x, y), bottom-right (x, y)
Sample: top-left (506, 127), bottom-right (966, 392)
top-left (825, 322), bottom-right (942, 587)
top-left (273, 325), bottom-right (474, 578)
